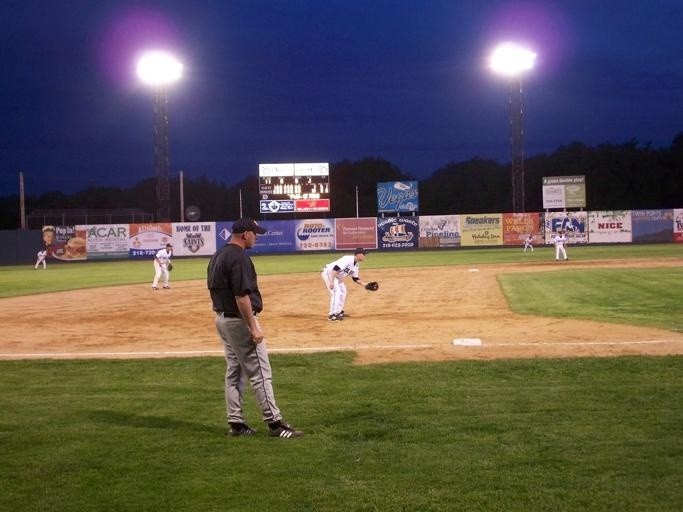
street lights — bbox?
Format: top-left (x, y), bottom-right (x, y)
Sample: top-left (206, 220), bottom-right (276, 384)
top-left (489, 43), bottom-right (537, 215)
top-left (135, 49), bottom-right (183, 221)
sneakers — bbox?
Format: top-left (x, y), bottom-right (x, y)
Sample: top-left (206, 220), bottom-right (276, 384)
top-left (152, 286), bottom-right (170, 290)
top-left (328, 313), bottom-right (345, 322)
top-left (555, 258), bottom-right (568, 262)
top-left (267, 422), bottom-right (305, 440)
top-left (230, 425), bottom-right (257, 437)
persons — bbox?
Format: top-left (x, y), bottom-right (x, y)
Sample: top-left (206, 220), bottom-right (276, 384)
top-left (320, 247), bottom-right (379, 321)
top-left (150, 243), bottom-right (173, 291)
top-left (204, 217), bottom-right (304, 441)
top-left (42, 225), bottom-right (55, 256)
top-left (34, 246), bottom-right (47, 270)
top-left (523, 235), bottom-right (533, 253)
top-left (553, 230), bottom-right (568, 261)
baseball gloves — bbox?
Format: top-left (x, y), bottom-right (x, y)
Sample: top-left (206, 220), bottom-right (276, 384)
top-left (168, 264), bottom-right (172, 271)
top-left (365, 282), bottom-right (378, 290)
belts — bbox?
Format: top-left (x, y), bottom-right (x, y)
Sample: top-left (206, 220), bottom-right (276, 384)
top-left (215, 309), bottom-right (257, 318)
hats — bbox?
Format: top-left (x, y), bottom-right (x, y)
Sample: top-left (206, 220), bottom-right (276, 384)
top-left (166, 244), bottom-right (173, 248)
top-left (230, 217), bottom-right (267, 235)
top-left (355, 247), bottom-right (367, 254)
